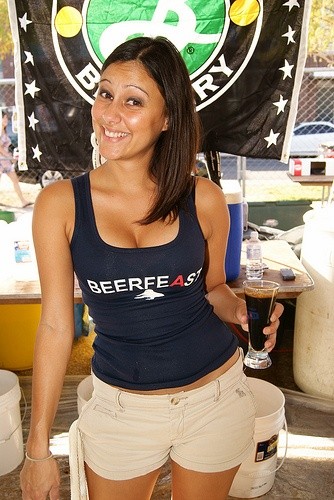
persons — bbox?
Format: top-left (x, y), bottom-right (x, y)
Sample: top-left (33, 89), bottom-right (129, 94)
top-left (19, 36), bottom-right (284, 500)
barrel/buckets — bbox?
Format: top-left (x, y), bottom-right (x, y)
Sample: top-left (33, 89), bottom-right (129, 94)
top-left (292, 206), bottom-right (334, 399)
top-left (0, 369), bottom-right (27, 476)
top-left (228, 377), bottom-right (288, 499)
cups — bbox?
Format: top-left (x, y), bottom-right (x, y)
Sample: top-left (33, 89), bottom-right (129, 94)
top-left (243, 280), bottom-right (280, 370)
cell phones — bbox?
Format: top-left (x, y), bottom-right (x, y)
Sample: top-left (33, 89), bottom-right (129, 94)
top-left (280, 268), bottom-right (296, 281)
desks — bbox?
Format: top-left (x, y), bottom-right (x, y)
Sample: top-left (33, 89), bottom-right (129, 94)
top-left (0, 240), bottom-right (315, 305)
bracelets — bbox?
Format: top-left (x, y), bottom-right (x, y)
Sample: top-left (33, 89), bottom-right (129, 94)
top-left (22, 451), bottom-right (56, 464)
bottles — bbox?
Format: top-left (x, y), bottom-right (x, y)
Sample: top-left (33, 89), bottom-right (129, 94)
top-left (246, 231), bottom-right (263, 281)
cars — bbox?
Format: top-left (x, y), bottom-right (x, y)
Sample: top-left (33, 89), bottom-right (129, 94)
top-left (15, 157), bottom-right (84, 189)
top-left (290, 122), bottom-right (334, 156)
